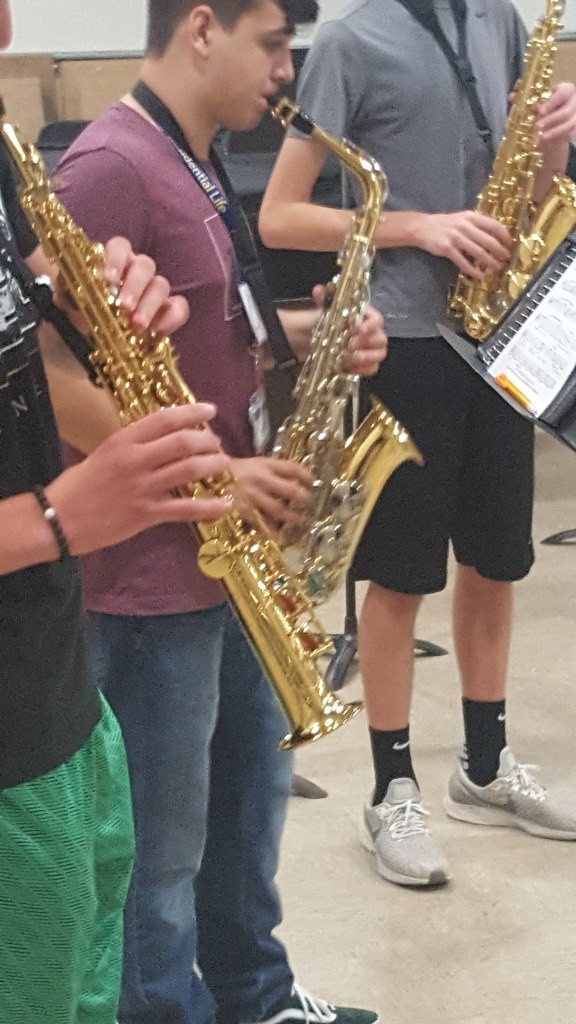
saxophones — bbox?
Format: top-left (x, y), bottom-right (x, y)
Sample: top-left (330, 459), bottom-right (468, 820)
top-left (445, 0), bottom-right (575, 345)
top-left (246, 83), bottom-right (428, 610)
top-left (0, 117), bottom-right (366, 754)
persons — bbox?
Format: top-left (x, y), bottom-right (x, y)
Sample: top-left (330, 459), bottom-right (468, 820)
top-left (55, 1), bottom-right (387, 1024)
top-left (257, 0), bottom-right (576, 884)
top-left (1, 0), bottom-right (231, 1024)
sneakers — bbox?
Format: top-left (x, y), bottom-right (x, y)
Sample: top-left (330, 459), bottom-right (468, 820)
top-left (442, 744), bottom-right (576, 841)
top-left (262, 982), bottom-right (378, 1023)
top-left (360, 774), bottom-right (450, 887)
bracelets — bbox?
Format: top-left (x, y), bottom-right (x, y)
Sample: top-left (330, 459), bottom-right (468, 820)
top-left (31, 485), bottom-right (70, 566)
